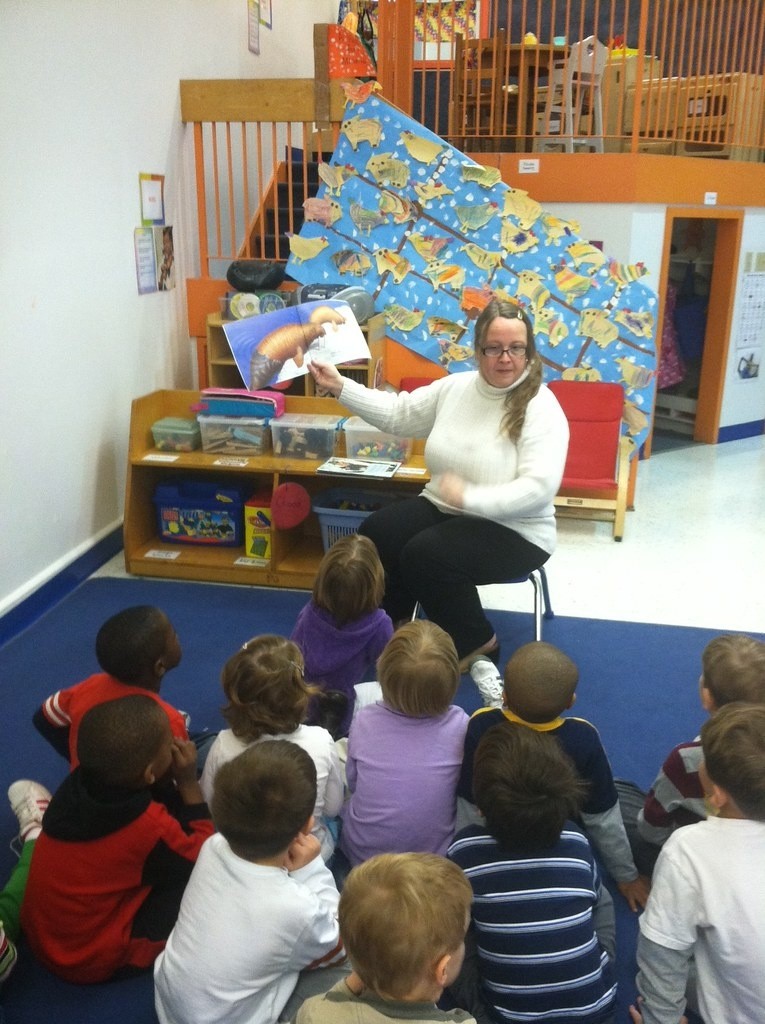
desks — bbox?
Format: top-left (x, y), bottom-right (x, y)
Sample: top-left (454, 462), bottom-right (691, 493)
top-left (466, 41), bottom-right (573, 151)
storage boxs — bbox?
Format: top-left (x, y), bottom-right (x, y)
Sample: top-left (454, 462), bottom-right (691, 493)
top-left (243, 481), bottom-right (274, 558)
top-left (269, 413), bottom-right (348, 459)
top-left (155, 472), bottom-right (248, 548)
top-left (197, 413), bottom-right (269, 455)
top-left (341, 416), bottom-right (414, 464)
top-left (150, 416), bottom-right (200, 452)
top-left (312, 490), bottom-right (418, 558)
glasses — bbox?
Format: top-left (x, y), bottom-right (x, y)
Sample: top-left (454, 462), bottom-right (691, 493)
top-left (481, 345), bottom-right (529, 359)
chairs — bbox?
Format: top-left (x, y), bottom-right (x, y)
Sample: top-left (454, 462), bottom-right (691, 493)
top-left (539, 35), bottom-right (608, 153)
top-left (452, 30), bottom-right (531, 150)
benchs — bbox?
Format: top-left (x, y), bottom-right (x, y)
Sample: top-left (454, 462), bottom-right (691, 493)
top-left (398, 376), bottom-right (634, 542)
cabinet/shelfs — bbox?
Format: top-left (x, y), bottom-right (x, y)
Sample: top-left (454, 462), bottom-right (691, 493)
top-left (123, 388), bottom-right (431, 591)
top-left (206, 312), bottom-right (386, 396)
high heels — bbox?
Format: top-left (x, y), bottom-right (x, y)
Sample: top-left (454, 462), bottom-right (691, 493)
top-left (458, 638), bottom-right (500, 671)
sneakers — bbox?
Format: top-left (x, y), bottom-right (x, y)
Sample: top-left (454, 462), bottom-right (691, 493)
top-left (7, 779), bottom-right (53, 838)
top-left (468, 656), bottom-right (504, 709)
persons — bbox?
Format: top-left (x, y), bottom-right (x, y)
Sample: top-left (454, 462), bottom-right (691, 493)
top-left (307, 299), bottom-right (569, 674)
top-left (614, 634), bottom-right (765, 1024)
top-left (287, 533), bottom-right (396, 739)
top-left (0, 604), bottom-right (652, 1024)
